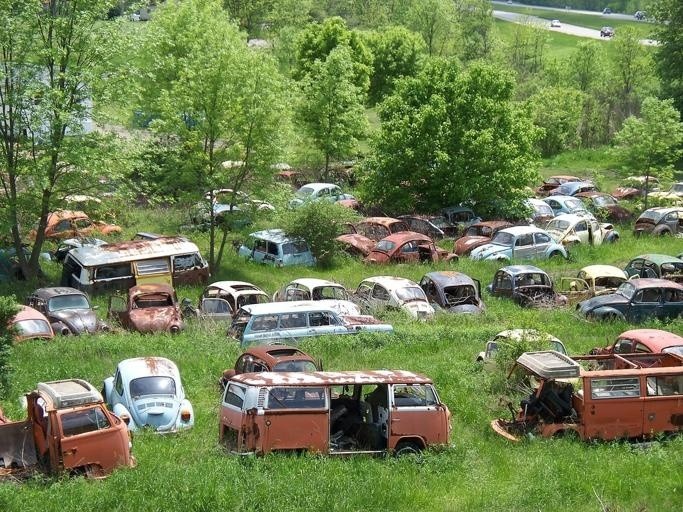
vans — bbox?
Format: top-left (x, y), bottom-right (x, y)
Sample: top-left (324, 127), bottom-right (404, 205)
top-left (218, 368), bottom-right (452, 463)
top-left (61, 235), bottom-right (212, 298)
top-left (239, 228), bottom-right (317, 269)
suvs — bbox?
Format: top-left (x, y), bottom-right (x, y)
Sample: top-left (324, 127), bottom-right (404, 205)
top-left (228, 300), bottom-right (393, 346)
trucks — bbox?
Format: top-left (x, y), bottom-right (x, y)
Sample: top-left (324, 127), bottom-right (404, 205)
top-left (491, 350), bottom-right (683, 443)
top-left (0, 377), bottom-right (139, 481)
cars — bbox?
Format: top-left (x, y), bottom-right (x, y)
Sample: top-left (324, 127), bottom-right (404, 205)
top-left (346, 275), bottom-right (436, 323)
top-left (59, 194), bottom-right (116, 224)
top-left (42, 237), bottom-right (108, 263)
top-left (416, 270), bottom-right (487, 318)
top-left (559, 264), bottom-right (643, 303)
top-left (537, 175), bottom-right (582, 195)
top-left (29, 286), bottom-right (112, 335)
top-left (487, 264), bottom-right (567, 309)
top-left (106, 281), bottom-right (186, 335)
top-left (548, 181), bottom-right (597, 196)
top-left (453, 220), bottom-right (515, 257)
top-left (363, 231), bottom-right (459, 265)
top-left (286, 182), bottom-right (356, 212)
top-left (633, 206), bottom-right (683, 238)
top-left (273, 277), bottom-right (361, 319)
top-left (541, 195), bottom-right (597, 222)
top-left (546, 214), bottom-right (620, 248)
top-left (525, 198), bottom-right (554, 222)
top-left (443, 206), bottom-right (482, 229)
top-left (476, 328), bottom-right (585, 388)
top-left (189, 188), bottom-right (276, 225)
top-left (647, 182), bottom-right (683, 205)
top-left (330, 219), bottom-right (377, 259)
top-left (591, 327), bottom-right (683, 369)
top-left (7, 305), bottom-right (55, 344)
top-left (353, 216), bottom-right (411, 241)
top-left (573, 191), bottom-right (631, 223)
top-left (575, 277), bottom-right (683, 324)
top-left (469, 225), bottom-right (568, 264)
top-left (623, 252), bottom-right (683, 282)
top-left (218, 345), bottom-right (340, 400)
top-left (611, 175), bottom-right (661, 201)
top-left (198, 279), bottom-right (275, 321)
top-left (395, 214), bottom-right (458, 239)
top-left (103, 356), bottom-right (194, 436)
top-left (274, 170), bottom-right (310, 189)
top-left (28, 208), bottom-right (122, 242)
